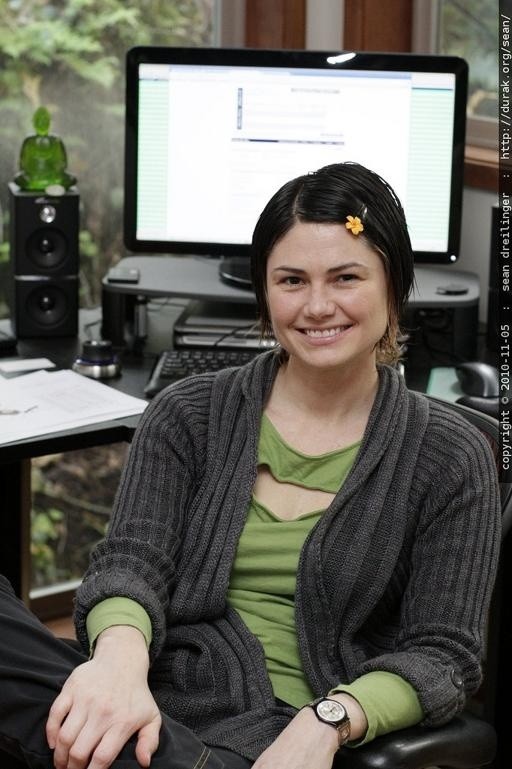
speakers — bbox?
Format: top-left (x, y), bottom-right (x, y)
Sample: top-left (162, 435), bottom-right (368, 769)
top-left (9, 183), bottom-right (81, 344)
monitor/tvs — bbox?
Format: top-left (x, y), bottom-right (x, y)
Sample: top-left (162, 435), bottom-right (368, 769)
top-left (124, 46), bottom-right (469, 288)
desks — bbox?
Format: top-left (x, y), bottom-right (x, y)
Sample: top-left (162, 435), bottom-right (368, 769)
top-left (0, 251), bottom-right (511, 638)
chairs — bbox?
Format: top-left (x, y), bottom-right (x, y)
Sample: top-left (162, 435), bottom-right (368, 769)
top-left (330, 387), bottom-right (508, 769)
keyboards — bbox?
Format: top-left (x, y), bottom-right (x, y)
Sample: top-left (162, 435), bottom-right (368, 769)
top-left (144, 349), bottom-right (263, 398)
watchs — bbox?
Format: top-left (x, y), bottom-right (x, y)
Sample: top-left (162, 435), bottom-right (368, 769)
top-left (301, 696), bottom-right (353, 749)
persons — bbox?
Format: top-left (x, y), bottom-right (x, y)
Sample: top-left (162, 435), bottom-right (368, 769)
top-left (14, 105), bottom-right (78, 192)
top-left (1, 159), bottom-right (505, 768)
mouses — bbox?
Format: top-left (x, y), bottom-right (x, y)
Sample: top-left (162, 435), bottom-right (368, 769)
top-left (455, 361), bottom-right (502, 399)
